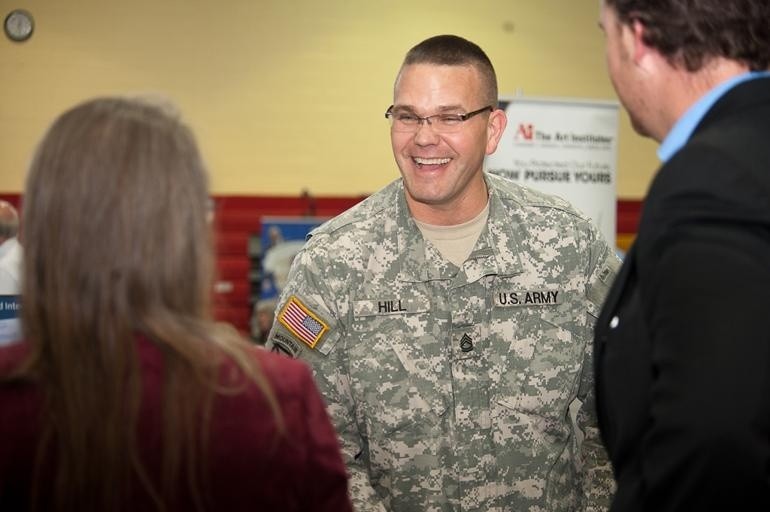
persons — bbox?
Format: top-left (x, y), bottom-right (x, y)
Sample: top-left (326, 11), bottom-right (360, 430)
top-left (0, 199), bottom-right (27, 347)
top-left (594, 0), bottom-right (769, 510)
top-left (266, 33), bottom-right (623, 511)
top-left (262, 225), bottom-right (312, 289)
top-left (0, 94), bottom-right (357, 511)
top-left (251, 298), bottom-right (279, 339)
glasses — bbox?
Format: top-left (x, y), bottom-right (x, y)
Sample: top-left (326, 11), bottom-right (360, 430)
top-left (385, 105), bottom-right (493, 131)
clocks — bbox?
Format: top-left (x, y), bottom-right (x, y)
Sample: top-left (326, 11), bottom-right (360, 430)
top-left (4, 9), bottom-right (34, 41)
